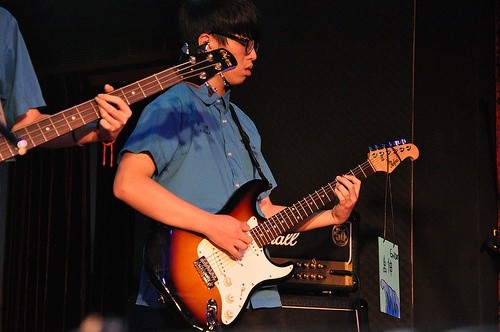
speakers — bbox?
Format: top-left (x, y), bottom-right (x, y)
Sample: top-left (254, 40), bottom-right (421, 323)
top-left (224, 289), bottom-right (368, 332)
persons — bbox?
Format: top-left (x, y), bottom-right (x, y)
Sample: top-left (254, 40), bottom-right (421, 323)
top-left (0, 1), bottom-right (136, 163)
top-left (112, 0), bottom-right (364, 332)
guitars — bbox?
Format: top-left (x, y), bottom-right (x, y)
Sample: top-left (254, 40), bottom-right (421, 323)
top-left (1, 42), bottom-right (238, 164)
top-left (148, 137), bottom-right (420, 332)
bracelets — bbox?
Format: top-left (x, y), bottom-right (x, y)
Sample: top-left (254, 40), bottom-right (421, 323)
top-left (71, 128), bottom-right (86, 148)
top-left (97, 127), bottom-right (116, 166)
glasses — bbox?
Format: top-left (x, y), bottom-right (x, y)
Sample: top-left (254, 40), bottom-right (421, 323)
top-left (206, 30), bottom-right (260, 54)
